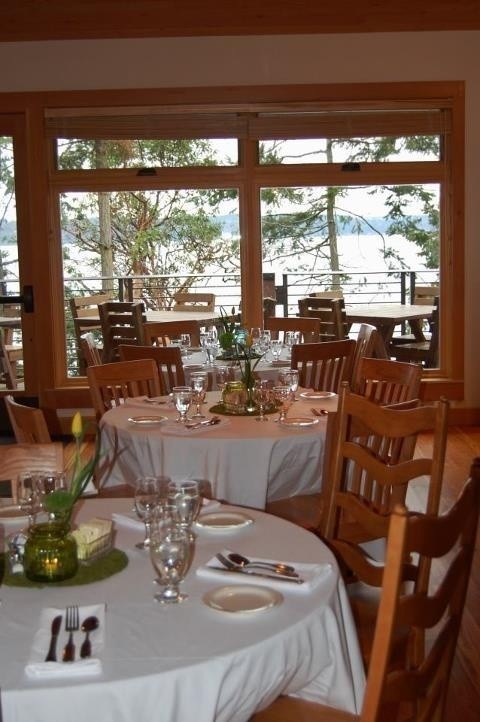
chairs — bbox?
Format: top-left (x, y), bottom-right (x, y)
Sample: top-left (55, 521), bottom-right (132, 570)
top-left (249, 458), bottom-right (480, 722)
top-left (171, 294), bottom-right (215, 330)
top-left (5, 394), bottom-right (52, 447)
top-left (266, 316), bottom-right (319, 345)
top-left (289, 340), bottom-right (355, 393)
top-left (394, 295), bottom-right (439, 368)
top-left (310, 291), bottom-right (345, 307)
top-left (94, 390), bottom-right (340, 513)
top-left (356, 324), bottom-right (376, 372)
top-left (99, 301), bottom-right (146, 360)
top-left (80, 332), bottom-right (100, 368)
top-left (298, 296), bottom-right (348, 340)
top-left (120, 343), bottom-right (184, 395)
top-left (320, 380), bottom-right (449, 671)
top-left (392, 286), bottom-right (440, 342)
top-left (267, 400), bottom-right (421, 533)
top-left (73, 295), bottom-right (107, 371)
top-left (350, 356), bottom-right (421, 402)
top-left (239, 297), bottom-right (275, 321)
top-left (87, 357), bottom-right (160, 409)
top-left (1, 303), bottom-right (25, 388)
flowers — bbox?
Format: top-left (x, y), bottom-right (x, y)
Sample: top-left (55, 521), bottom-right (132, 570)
top-left (39, 410), bottom-right (100, 526)
top-left (219, 334), bottom-right (270, 407)
top-left (217, 305), bottom-right (237, 355)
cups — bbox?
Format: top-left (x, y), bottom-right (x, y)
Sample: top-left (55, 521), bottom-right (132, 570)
top-left (177, 391), bottom-right (193, 424)
top-left (284, 370), bottom-right (299, 393)
top-left (190, 371), bottom-right (208, 399)
top-left (136, 474), bottom-right (169, 552)
top-left (285, 331), bottom-right (294, 351)
top-left (270, 339), bottom-right (283, 361)
top-left (191, 378), bottom-right (205, 419)
top-left (250, 378), bottom-right (271, 423)
top-left (250, 328), bottom-right (260, 345)
top-left (173, 387), bottom-right (194, 425)
top-left (180, 334), bottom-right (191, 362)
top-left (150, 502), bottom-right (196, 606)
top-left (272, 386), bottom-right (295, 424)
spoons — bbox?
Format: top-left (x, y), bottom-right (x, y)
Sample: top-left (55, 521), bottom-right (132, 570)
top-left (79, 615), bottom-right (99, 659)
top-left (226, 553), bottom-right (294, 573)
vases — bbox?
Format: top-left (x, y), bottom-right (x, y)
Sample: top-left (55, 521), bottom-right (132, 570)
top-left (241, 361), bottom-right (258, 411)
top-left (25, 524), bottom-right (78, 581)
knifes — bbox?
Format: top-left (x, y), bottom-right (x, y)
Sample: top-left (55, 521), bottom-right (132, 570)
top-left (204, 565), bottom-right (305, 584)
top-left (44, 614), bottom-right (62, 663)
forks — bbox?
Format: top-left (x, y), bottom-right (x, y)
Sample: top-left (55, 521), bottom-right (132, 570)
top-left (311, 408), bottom-right (328, 417)
top-left (62, 604), bottom-right (79, 664)
top-left (213, 552), bottom-right (298, 577)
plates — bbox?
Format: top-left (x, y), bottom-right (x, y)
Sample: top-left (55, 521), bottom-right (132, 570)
top-left (197, 511), bottom-right (253, 532)
top-left (300, 391), bottom-right (336, 400)
top-left (127, 415), bottom-right (169, 425)
top-left (0, 504), bottom-right (47, 523)
top-left (202, 584), bottom-right (284, 615)
top-left (275, 417), bottom-right (319, 428)
